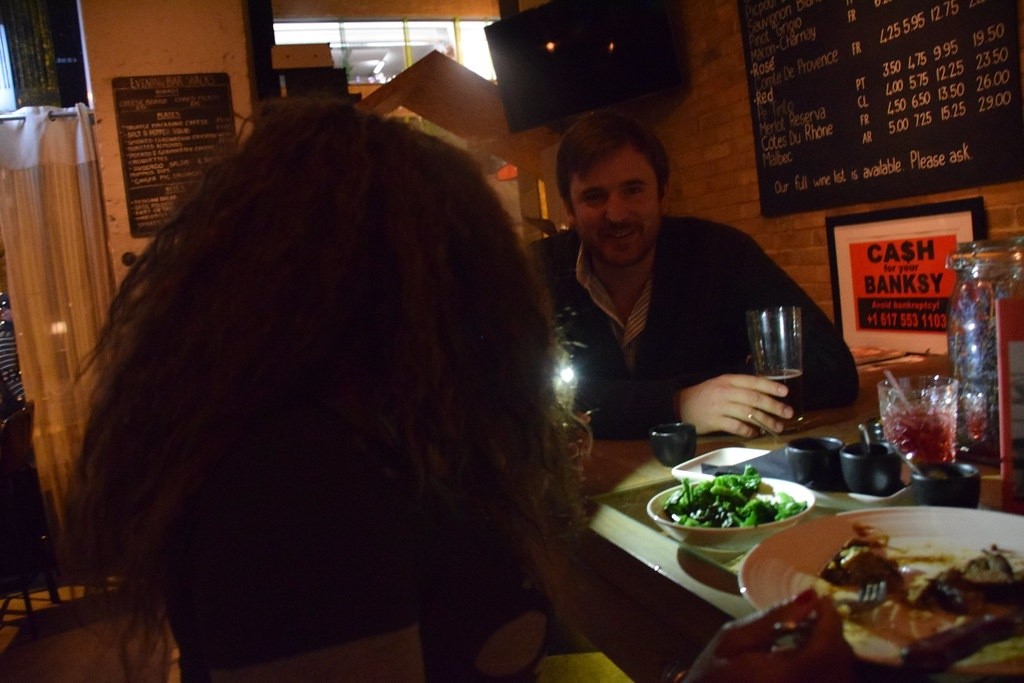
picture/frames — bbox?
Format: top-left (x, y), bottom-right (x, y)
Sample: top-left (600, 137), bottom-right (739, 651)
top-left (825, 193), bottom-right (988, 354)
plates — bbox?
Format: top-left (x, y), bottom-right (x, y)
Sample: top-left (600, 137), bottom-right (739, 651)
top-left (647, 474), bottom-right (816, 555)
top-left (671, 446), bottom-right (914, 510)
top-left (739, 506), bottom-right (1024, 683)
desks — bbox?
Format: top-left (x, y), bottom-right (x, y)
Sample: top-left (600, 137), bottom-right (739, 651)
top-left (585, 354), bottom-right (1023, 646)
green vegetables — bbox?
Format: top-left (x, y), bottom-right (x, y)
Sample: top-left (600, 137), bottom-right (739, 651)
top-left (664, 465), bottom-right (808, 527)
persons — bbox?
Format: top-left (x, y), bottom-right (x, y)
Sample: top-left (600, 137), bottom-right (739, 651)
top-left (0, 294), bottom-right (26, 415)
top-left (527, 111), bottom-right (858, 439)
top-left (61, 104), bottom-right (860, 683)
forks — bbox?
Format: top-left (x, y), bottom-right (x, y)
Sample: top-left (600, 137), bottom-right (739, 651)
top-left (771, 578), bottom-right (888, 637)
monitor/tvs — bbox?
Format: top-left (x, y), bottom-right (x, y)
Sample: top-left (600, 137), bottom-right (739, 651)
top-left (485, 1), bottom-right (686, 133)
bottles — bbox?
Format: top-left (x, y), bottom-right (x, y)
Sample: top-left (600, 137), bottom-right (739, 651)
top-left (947, 235), bottom-right (1024, 466)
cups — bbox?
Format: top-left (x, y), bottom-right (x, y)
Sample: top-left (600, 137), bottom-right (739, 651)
top-left (840, 440), bottom-right (903, 496)
top-left (876, 376), bottom-right (957, 467)
top-left (786, 435), bottom-right (845, 492)
top-left (745, 305), bottom-right (805, 423)
top-left (648, 422), bottom-right (697, 467)
top-left (912, 463), bottom-right (979, 510)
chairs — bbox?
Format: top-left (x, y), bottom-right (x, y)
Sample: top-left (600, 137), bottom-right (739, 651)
top-left (0, 400), bottom-right (57, 637)
